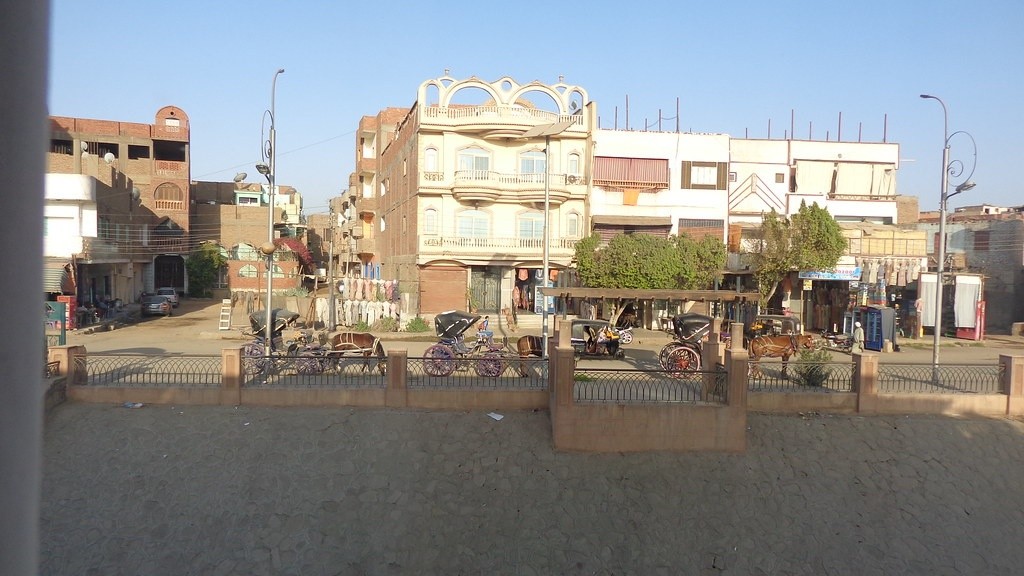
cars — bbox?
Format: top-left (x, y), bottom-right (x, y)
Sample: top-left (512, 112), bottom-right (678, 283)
top-left (141, 296), bottom-right (172, 317)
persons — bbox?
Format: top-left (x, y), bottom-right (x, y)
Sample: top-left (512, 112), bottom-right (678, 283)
top-left (848, 321), bottom-right (864, 356)
top-left (479, 316), bottom-right (489, 343)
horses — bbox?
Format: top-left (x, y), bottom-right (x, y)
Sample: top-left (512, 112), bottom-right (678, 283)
top-left (328, 333), bottom-right (385, 375)
top-left (749, 334), bottom-right (813, 380)
top-left (518, 335), bottom-right (553, 379)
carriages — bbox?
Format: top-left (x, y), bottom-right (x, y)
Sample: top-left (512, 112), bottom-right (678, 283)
top-left (423, 310), bottom-right (581, 378)
top-left (239, 307), bottom-right (388, 376)
top-left (657, 312), bottom-right (815, 380)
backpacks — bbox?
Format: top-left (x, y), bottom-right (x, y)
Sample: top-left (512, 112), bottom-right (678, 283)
top-left (478, 321), bottom-right (485, 329)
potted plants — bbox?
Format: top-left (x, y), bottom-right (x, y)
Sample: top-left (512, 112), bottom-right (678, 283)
top-left (793, 338), bottom-right (834, 385)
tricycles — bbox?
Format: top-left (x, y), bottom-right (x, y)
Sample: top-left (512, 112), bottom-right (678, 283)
top-left (750, 315), bottom-right (801, 337)
top-left (569, 319), bottom-right (627, 362)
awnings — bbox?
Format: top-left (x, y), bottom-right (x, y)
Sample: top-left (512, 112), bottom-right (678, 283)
top-left (43, 256), bottom-right (75, 293)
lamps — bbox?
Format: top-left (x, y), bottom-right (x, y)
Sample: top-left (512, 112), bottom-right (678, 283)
top-left (104, 152), bottom-right (115, 164)
top-left (80, 141), bottom-right (88, 152)
top-left (132, 187), bottom-right (141, 201)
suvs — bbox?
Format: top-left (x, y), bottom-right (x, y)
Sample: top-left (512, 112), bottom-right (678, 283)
top-left (156, 287), bottom-right (179, 307)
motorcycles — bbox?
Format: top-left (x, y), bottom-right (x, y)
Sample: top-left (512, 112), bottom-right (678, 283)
top-left (614, 327), bottom-right (634, 344)
top-left (812, 330), bottom-right (855, 353)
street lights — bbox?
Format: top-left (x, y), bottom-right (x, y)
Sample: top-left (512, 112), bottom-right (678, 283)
top-left (919, 93), bottom-right (978, 387)
top-left (254, 68), bottom-right (286, 359)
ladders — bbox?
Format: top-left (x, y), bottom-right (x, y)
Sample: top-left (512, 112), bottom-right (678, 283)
top-left (219, 307), bottom-right (231, 331)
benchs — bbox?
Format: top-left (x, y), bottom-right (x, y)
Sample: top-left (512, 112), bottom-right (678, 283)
top-left (452, 336), bottom-right (470, 354)
top-left (271, 333), bottom-right (286, 352)
